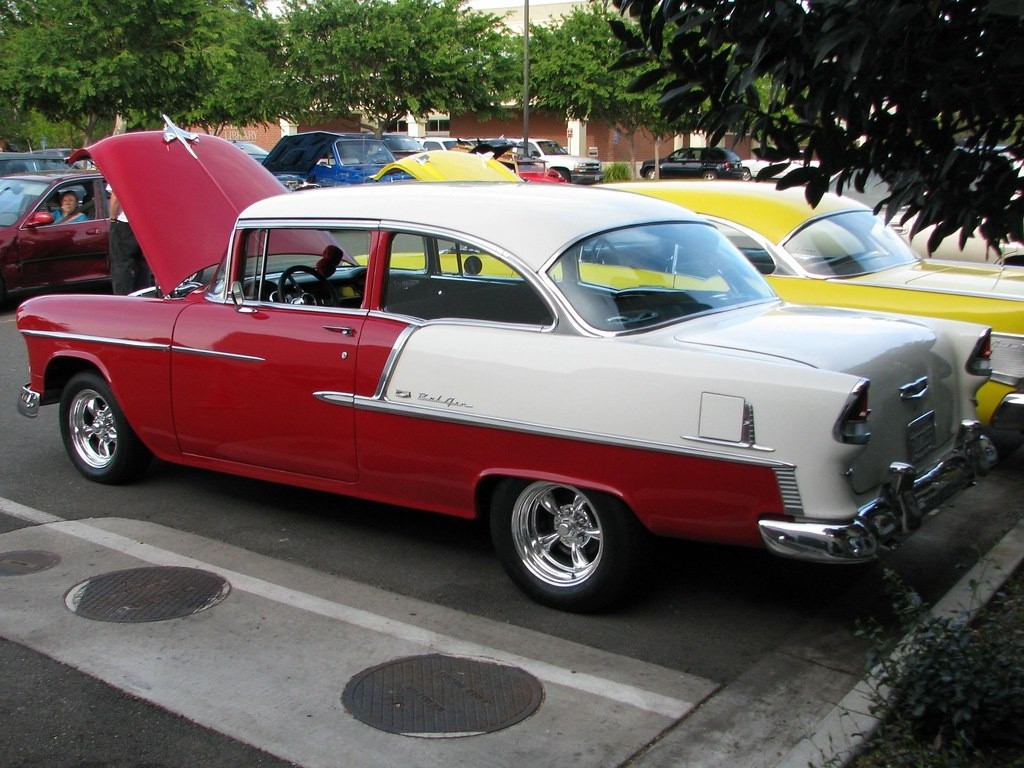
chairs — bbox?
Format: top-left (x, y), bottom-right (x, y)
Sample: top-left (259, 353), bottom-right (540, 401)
top-left (82, 194), bottom-right (95, 220)
top-left (43, 192), bottom-right (60, 212)
top-left (338, 267), bottom-right (401, 309)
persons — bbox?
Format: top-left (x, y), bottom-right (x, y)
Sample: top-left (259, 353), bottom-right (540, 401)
top-left (105, 160), bottom-right (152, 298)
top-left (39, 190), bottom-right (91, 227)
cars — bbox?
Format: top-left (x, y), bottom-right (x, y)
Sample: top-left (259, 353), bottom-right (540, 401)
top-left (740, 149), bottom-right (844, 184)
top-left (639, 148), bottom-right (741, 181)
top-left (13, 114), bottom-right (998, 616)
top-left (1, 124), bottom-right (604, 306)
top-left (338, 148), bottom-right (1024, 450)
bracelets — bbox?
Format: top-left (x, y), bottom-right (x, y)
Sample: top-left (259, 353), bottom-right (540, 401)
top-left (109, 217), bottom-right (119, 222)
